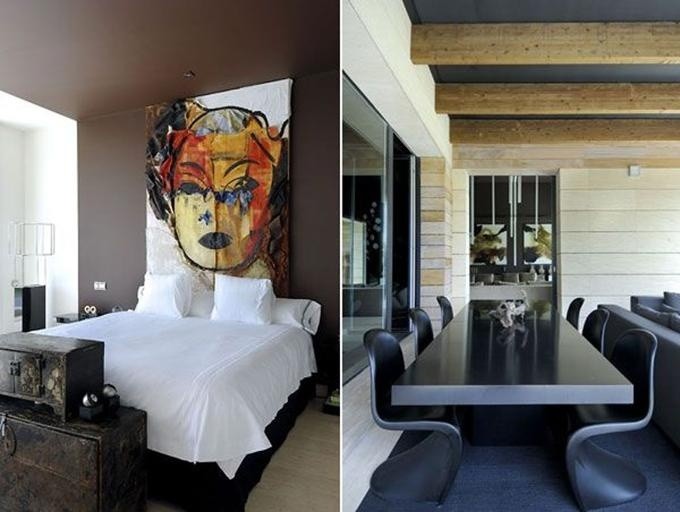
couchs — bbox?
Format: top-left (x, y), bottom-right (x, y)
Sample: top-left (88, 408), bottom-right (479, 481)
top-left (598, 303), bottom-right (680, 450)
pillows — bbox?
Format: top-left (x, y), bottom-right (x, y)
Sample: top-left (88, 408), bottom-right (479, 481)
top-left (135, 274), bottom-right (191, 317)
top-left (137, 287), bottom-right (321, 335)
top-left (470, 272), bottom-right (545, 285)
top-left (633, 292), bottom-right (680, 331)
top-left (211, 273), bottom-right (273, 325)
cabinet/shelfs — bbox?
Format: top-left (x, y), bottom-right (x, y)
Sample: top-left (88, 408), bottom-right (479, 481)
top-left (0, 395), bottom-right (149, 512)
top-left (1, 333), bottom-right (104, 422)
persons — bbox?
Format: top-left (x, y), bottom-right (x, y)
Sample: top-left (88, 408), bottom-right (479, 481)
top-left (144, 96), bottom-right (291, 296)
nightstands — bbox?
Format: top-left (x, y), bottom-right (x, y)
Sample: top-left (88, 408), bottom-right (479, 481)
top-left (52, 312), bottom-right (101, 327)
top-left (318, 319), bottom-right (339, 416)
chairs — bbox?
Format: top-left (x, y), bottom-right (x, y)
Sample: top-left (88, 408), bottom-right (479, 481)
top-left (364, 329), bottom-right (462, 506)
top-left (582, 308), bottom-right (610, 354)
top-left (565, 328), bottom-right (657, 511)
top-left (437, 296), bottom-right (453, 330)
top-left (566, 298), bottom-right (585, 330)
top-left (410, 310), bottom-right (434, 360)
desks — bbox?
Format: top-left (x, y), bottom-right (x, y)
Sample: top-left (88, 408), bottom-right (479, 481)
top-left (391, 299), bottom-right (633, 447)
top-left (343, 287), bottom-right (383, 316)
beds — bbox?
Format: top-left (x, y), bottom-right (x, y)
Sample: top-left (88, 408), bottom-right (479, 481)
top-left (27, 311), bottom-right (317, 512)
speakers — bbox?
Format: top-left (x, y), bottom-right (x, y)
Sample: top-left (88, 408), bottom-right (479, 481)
top-left (79, 305), bottom-right (99, 319)
top-left (79, 384), bottom-right (122, 423)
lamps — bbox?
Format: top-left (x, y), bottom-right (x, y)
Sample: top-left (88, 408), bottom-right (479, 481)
top-left (12, 224), bottom-right (55, 286)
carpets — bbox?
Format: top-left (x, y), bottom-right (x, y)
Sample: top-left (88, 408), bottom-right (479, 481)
top-left (356, 404), bottom-right (680, 512)
